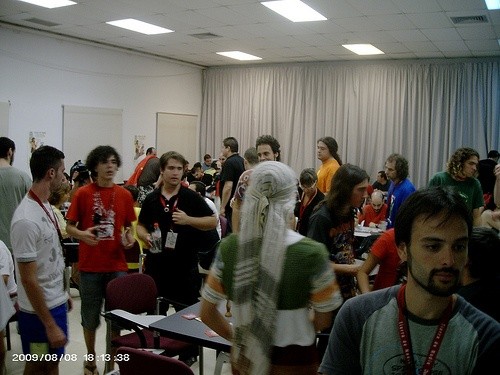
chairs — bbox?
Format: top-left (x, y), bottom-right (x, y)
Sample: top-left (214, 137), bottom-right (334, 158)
top-left (99, 274), bottom-right (200, 375)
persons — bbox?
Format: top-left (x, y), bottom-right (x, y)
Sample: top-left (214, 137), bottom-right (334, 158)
top-left (65, 145), bottom-right (138, 375)
top-left (12, 145), bottom-right (73, 375)
top-left (317, 185), bottom-right (500, 375)
top-left (0, 133), bottom-right (499, 296)
top-left (0, 239), bottom-right (18, 307)
top-left (135, 151), bottom-right (219, 369)
top-left (198, 160), bottom-right (343, 375)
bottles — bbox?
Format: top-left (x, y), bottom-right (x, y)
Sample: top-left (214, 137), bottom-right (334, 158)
top-left (151, 223), bottom-right (162, 253)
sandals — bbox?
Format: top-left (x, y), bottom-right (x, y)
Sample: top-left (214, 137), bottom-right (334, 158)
top-left (84, 360), bottom-right (99, 375)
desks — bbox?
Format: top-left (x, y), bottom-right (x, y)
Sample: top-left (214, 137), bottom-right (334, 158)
top-left (149, 299), bottom-right (233, 375)
